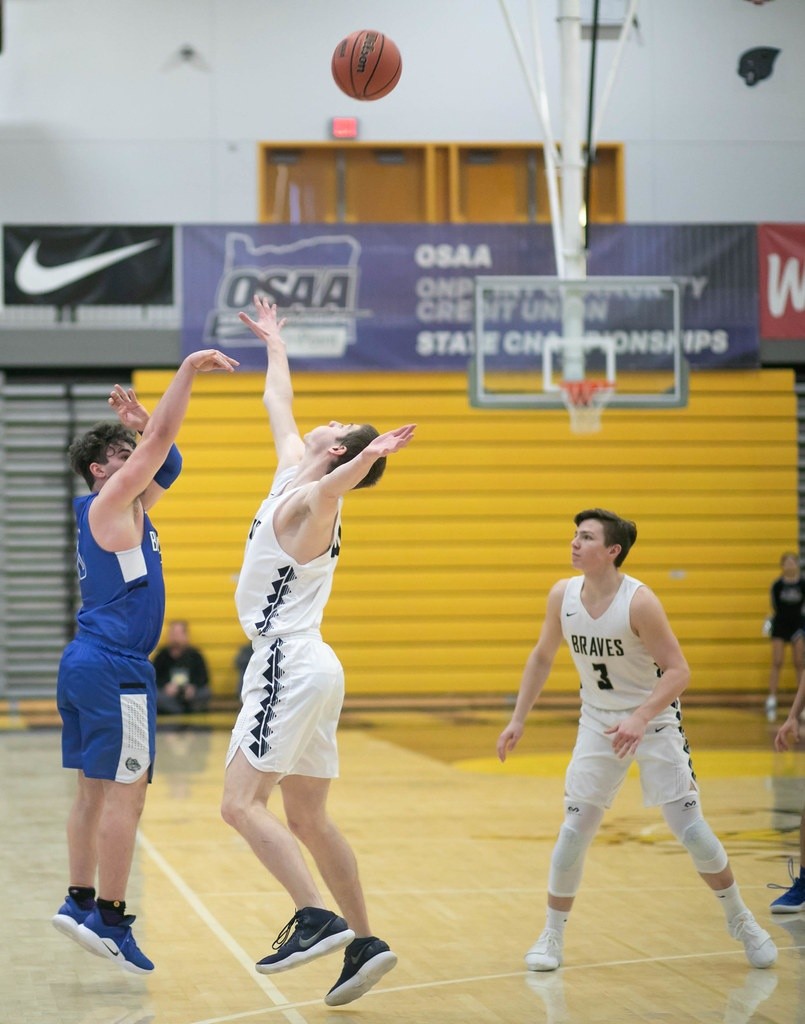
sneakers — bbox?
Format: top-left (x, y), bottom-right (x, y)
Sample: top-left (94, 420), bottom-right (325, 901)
top-left (51, 896), bottom-right (155, 976)
top-left (526, 924), bottom-right (566, 970)
top-left (256, 909), bottom-right (355, 974)
top-left (728, 912), bottom-right (777, 968)
top-left (769, 858), bottom-right (805, 912)
top-left (325, 936), bottom-right (397, 1006)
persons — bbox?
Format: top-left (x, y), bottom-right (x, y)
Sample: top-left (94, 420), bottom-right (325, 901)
top-left (492, 509), bottom-right (779, 973)
top-left (765, 668), bottom-right (805, 915)
top-left (148, 621), bottom-right (212, 715)
top-left (763, 548), bottom-right (805, 721)
top-left (231, 638), bottom-right (252, 711)
top-left (48, 348), bottom-right (237, 981)
top-left (217, 291), bottom-right (420, 1007)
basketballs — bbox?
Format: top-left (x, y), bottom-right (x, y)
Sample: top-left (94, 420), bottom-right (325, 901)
top-left (331, 28), bottom-right (403, 101)
